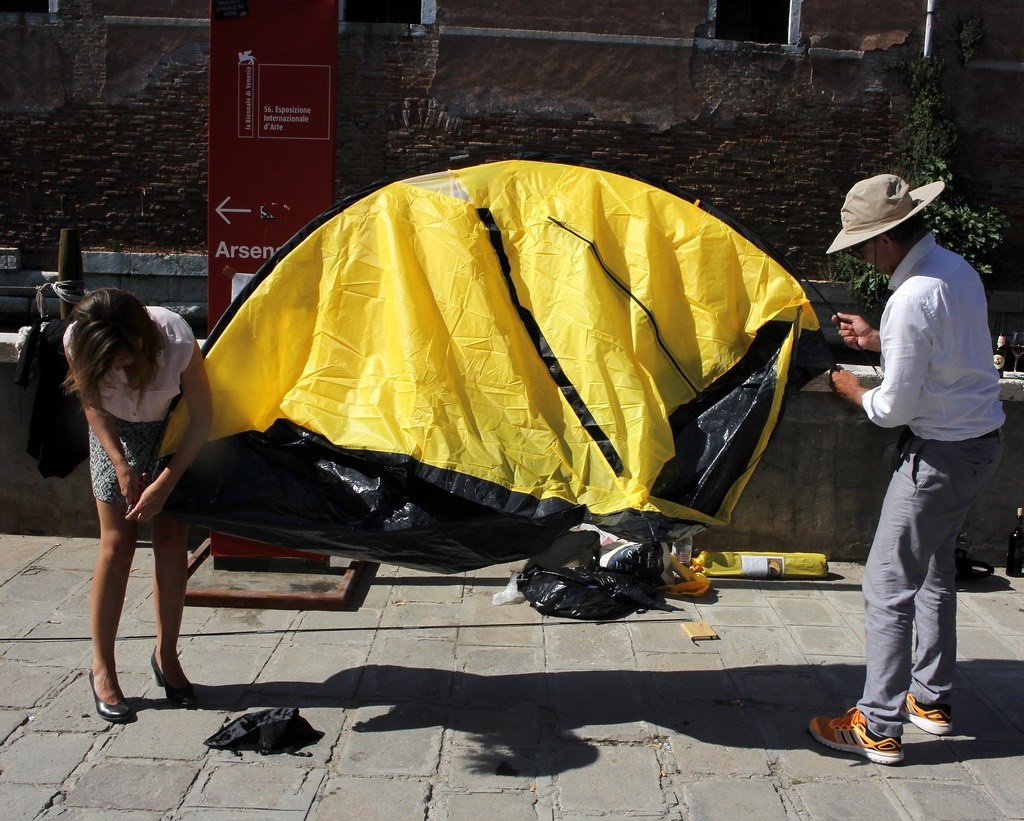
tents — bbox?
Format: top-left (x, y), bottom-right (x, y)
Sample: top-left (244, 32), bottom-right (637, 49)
top-left (125, 157), bottom-right (879, 574)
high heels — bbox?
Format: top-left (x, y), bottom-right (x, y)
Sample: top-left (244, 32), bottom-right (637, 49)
top-left (87, 670), bottom-right (131, 720)
top-left (152, 646), bottom-right (193, 707)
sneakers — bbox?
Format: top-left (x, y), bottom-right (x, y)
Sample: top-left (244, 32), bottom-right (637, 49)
top-left (810, 706), bottom-right (905, 763)
top-left (902, 692), bottom-right (953, 734)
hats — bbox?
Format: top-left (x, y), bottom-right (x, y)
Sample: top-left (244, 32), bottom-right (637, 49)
top-left (826, 174), bottom-right (945, 256)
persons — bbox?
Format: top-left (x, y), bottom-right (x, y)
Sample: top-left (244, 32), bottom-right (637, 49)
top-left (806, 171), bottom-right (1003, 766)
top-left (61, 288), bottom-right (213, 727)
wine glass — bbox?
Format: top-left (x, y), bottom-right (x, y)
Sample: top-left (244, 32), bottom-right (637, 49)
top-left (1009, 331), bottom-right (1024, 376)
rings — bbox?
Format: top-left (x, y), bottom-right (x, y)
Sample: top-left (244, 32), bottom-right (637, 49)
top-left (139, 513), bottom-right (146, 520)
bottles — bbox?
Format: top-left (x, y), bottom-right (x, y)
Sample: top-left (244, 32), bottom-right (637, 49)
top-left (1006, 508), bottom-right (1024, 576)
top-left (993, 332), bottom-right (1006, 378)
